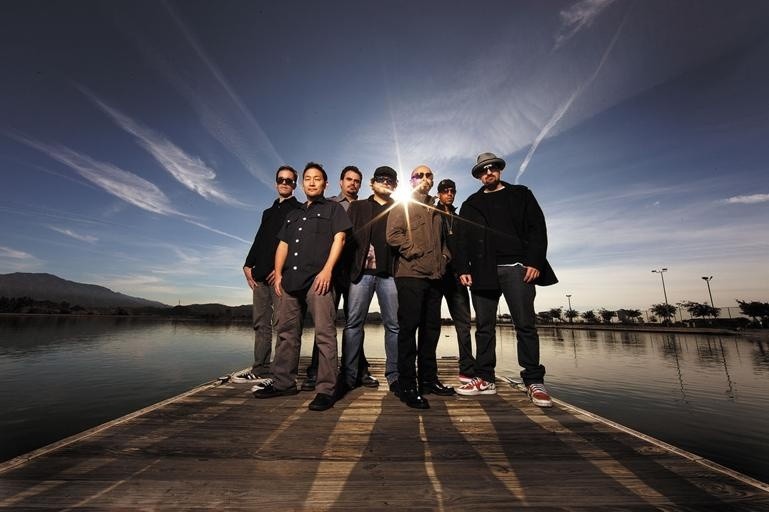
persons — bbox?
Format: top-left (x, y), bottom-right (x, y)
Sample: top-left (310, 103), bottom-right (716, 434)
top-left (437, 180), bottom-right (476, 381)
top-left (233, 167), bottom-right (301, 392)
top-left (388, 164), bottom-right (455, 410)
top-left (252, 166), bottom-right (356, 411)
top-left (300, 169), bottom-right (379, 390)
top-left (455, 153), bottom-right (560, 406)
top-left (336, 166), bottom-right (403, 398)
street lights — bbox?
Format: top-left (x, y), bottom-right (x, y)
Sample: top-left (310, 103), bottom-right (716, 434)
top-left (668, 334), bottom-right (689, 407)
top-left (565, 294), bottom-right (573, 310)
top-left (716, 336), bottom-right (737, 402)
top-left (702, 276), bottom-right (717, 318)
top-left (570, 329), bottom-right (578, 361)
top-left (652, 265), bottom-right (673, 322)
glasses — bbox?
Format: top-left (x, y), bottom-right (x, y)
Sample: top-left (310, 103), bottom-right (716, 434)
top-left (277, 177), bottom-right (296, 186)
top-left (375, 176), bottom-right (396, 186)
top-left (441, 188), bottom-right (456, 194)
top-left (411, 173), bottom-right (433, 179)
top-left (475, 162), bottom-right (500, 179)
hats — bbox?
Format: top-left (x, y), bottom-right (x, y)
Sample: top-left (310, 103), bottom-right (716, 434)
top-left (437, 178), bottom-right (455, 192)
top-left (374, 166), bottom-right (397, 181)
top-left (472, 152), bottom-right (506, 179)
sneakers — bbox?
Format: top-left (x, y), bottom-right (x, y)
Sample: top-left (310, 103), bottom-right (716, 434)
top-left (253, 382), bottom-right (298, 398)
top-left (231, 367), bottom-right (271, 384)
top-left (250, 378), bottom-right (276, 393)
top-left (526, 382), bottom-right (553, 408)
top-left (459, 371), bottom-right (480, 383)
top-left (453, 378), bottom-right (497, 395)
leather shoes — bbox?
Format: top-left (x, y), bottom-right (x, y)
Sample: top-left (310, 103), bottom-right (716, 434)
top-left (301, 373), bottom-right (358, 411)
top-left (388, 380), bottom-right (430, 409)
top-left (359, 373), bottom-right (380, 388)
top-left (419, 378), bottom-right (455, 396)
